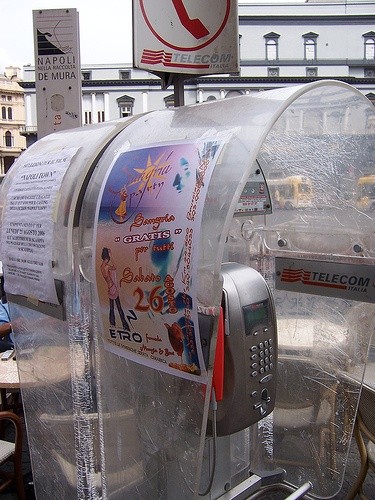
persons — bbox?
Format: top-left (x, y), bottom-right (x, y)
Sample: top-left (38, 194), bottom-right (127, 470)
top-left (0, 293), bottom-right (69, 348)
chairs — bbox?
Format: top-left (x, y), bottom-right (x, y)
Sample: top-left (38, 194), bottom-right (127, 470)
top-left (1, 302), bottom-right (374, 499)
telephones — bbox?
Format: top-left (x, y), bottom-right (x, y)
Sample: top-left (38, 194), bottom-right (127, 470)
top-left (164, 262), bottom-right (276, 436)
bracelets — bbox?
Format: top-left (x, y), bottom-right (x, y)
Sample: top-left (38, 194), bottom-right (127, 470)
top-left (9, 322), bottom-right (12, 334)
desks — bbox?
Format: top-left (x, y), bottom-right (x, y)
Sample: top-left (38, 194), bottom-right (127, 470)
top-left (275, 319), bottom-right (348, 355)
top-left (1, 346), bottom-right (84, 388)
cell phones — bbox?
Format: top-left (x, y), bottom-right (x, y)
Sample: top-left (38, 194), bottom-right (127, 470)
top-left (1, 350), bottom-right (14, 360)
